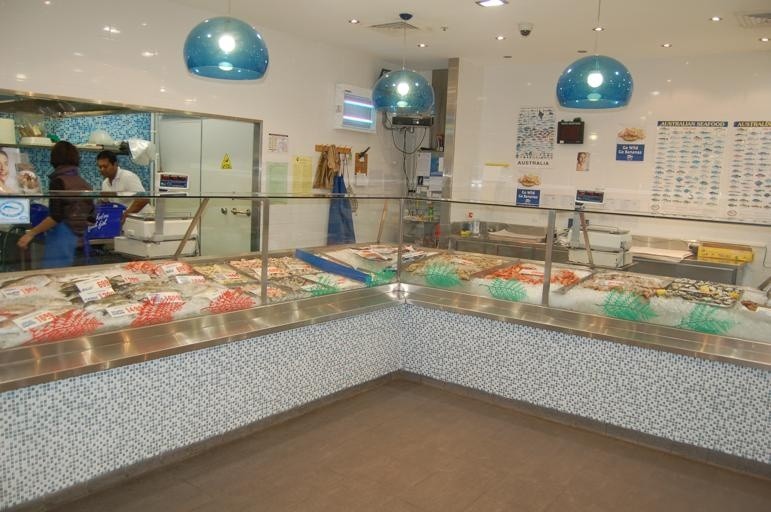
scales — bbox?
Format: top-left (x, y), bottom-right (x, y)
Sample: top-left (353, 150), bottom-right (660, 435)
top-left (566, 188), bottom-right (633, 251)
top-left (124, 171), bottom-right (198, 242)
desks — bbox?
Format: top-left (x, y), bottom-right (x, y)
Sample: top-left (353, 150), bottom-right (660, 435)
top-left (436, 219), bottom-right (745, 284)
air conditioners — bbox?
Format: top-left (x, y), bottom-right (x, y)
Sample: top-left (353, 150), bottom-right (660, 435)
top-left (383, 111), bottom-right (435, 129)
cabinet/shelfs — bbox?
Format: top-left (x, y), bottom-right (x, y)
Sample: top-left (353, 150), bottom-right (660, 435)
top-left (0, 89), bottom-right (159, 272)
top-left (402, 194), bottom-right (440, 245)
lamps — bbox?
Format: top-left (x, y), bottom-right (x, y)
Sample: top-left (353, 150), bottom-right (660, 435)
top-left (332, 83), bottom-right (379, 132)
top-left (372, 13), bottom-right (434, 111)
top-left (556, 0), bottom-right (634, 108)
top-left (184, 0), bottom-right (269, 81)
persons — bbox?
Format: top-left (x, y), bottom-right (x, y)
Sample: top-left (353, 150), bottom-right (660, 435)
top-left (16, 140), bottom-right (96, 269)
top-left (0, 151), bottom-right (18, 194)
top-left (96, 150), bottom-right (149, 225)
top-left (577, 152), bottom-right (587, 170)
